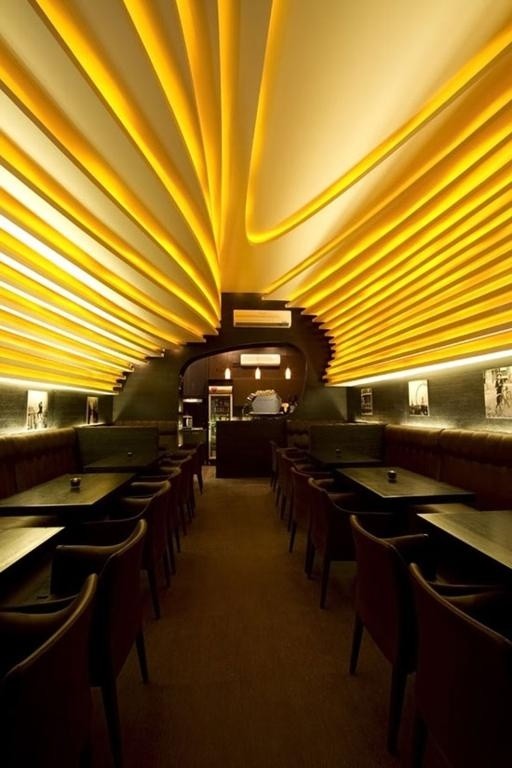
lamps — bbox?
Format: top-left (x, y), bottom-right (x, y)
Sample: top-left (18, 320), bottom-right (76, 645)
top-left (255, 347), bottom-right (261, 379)
top-left (285, 347), bottom-right (292, 380)
top-left (225, 351), bottom-right (231, 379)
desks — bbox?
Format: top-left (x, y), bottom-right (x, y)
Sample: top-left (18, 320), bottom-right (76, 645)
top-left (417, 510), bottom-right (512, 569)
top-left (1, 525), bottom-right (66, 574)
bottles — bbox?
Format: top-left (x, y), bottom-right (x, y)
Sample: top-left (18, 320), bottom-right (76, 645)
top-left (128, 448), bottom-right (133, 457)
top-left (70, 478), bottom-right (81, 488)
top-left (335, 447), bottom-right (341, 456)
top-left (386, 470), bottom-right (396, 482)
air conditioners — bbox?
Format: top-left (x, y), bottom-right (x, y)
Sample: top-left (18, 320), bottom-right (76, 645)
top-left (233, 308), bottom-right (291, 328)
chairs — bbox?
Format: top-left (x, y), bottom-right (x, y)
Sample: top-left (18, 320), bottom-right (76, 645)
top-left (1, 518), bottom-right (147, 767)
top-left (265, 418), bottom-right (511, 608)
top-left (406, 560), bottom-right (511, 765)
top-left (1, 420), bottom-right (206, 621)
top-left (1, 571), bottom-right (100, 767)
top-left (349, 514), bottom-right (505, 752)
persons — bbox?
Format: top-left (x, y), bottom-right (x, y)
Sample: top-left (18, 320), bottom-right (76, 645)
top-left (36, 401), bottom-right (43, 418)
top-left (494, 372), bottom-right (504, 413)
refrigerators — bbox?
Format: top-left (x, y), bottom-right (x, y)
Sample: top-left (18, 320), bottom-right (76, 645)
top-left (208, 385), bottom-right (235, 466)
